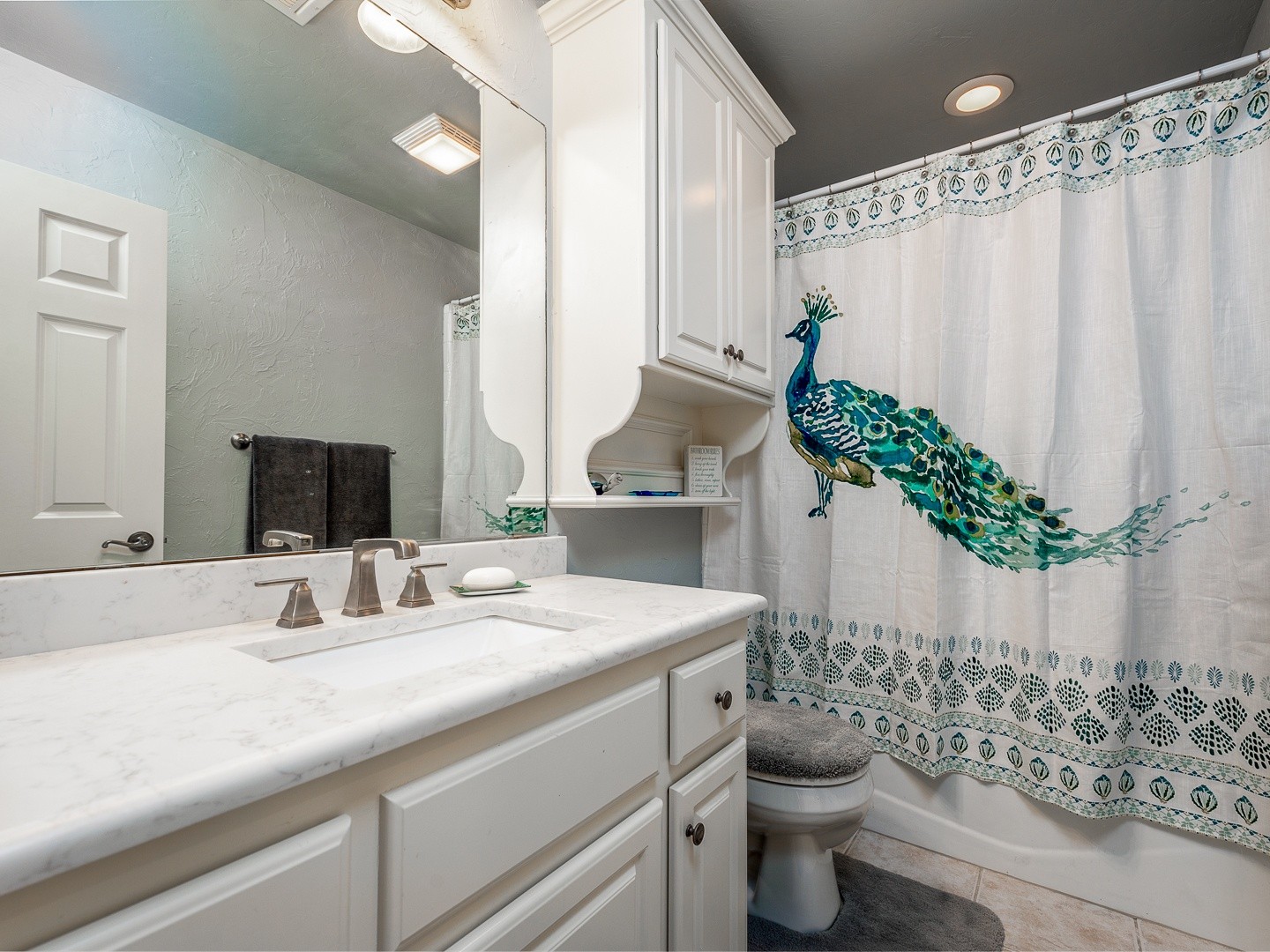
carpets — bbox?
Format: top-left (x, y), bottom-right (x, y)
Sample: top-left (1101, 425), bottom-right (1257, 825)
top-left (745, 841), bottom-right (1005, 952)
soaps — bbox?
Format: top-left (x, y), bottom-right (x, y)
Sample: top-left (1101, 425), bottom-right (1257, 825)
top-left (461, 568), bottom-right (518, 590)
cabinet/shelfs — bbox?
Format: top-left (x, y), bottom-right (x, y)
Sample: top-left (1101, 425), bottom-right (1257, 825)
top-left (25, 672), bottom-right (669, 952)
top-left (662, 640), bottom-right (747, 952)
top-left (539, 0), bottom-right (798, 516)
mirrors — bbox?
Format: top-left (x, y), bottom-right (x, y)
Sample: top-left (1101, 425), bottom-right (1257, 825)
top-left (1, 2), bottom-right (552, 575)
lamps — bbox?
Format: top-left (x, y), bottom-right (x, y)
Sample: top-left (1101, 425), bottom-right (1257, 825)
top-left (392, 113), bottom-right (482, 175)
top-left (357, 1), bottom-right (430, 55)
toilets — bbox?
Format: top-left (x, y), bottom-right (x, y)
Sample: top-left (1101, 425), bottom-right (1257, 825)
top-left (742, 698), bottom-right (873, 935)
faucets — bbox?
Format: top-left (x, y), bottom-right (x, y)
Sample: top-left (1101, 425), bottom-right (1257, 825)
top-left (340, 539), bottom-right (420, 618)
top-left (262, 529), bottom-right (314, 553)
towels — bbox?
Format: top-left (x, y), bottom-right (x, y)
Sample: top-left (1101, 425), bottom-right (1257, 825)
top-left (247, 434), bottom-right (327, 553)
top-left (325, 440), bottom-right (393, 549)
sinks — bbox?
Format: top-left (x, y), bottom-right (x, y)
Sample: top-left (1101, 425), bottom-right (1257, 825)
top-left (234, 597), bottom-right (614, 690)
